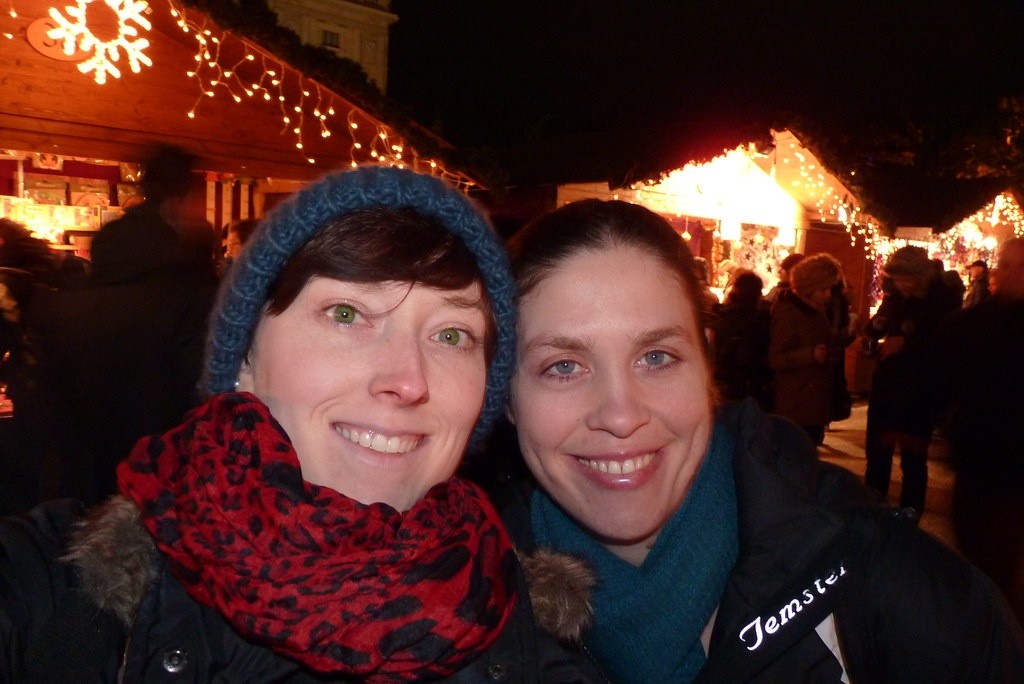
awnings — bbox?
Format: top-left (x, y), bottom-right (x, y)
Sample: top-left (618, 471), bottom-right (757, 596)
top-left (162, 0), bottom-right (526, 200)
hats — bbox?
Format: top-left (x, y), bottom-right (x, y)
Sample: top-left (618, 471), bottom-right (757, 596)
top-left (884, 246), bottom-right (928, 277)
top-left (198, 165), bottom-right (517, 472)
top-left (791, 254), bottom-right (843, 296)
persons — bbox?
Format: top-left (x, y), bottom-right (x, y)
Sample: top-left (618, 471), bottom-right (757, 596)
top-left (476, 200), bottom-right (1024, 684)
top-left (765, 253), bottom-right (841, 460)
top-left (12, 168), bottom-right (622, 684)
top-left (79, 142), bottom-right (200, 511)
top-left (953, 233), bottom-right (1023, 599)
top-left (862, 246), bottom-right (958, 523)
top-left (708, 272), bottom-right (779, 409)
